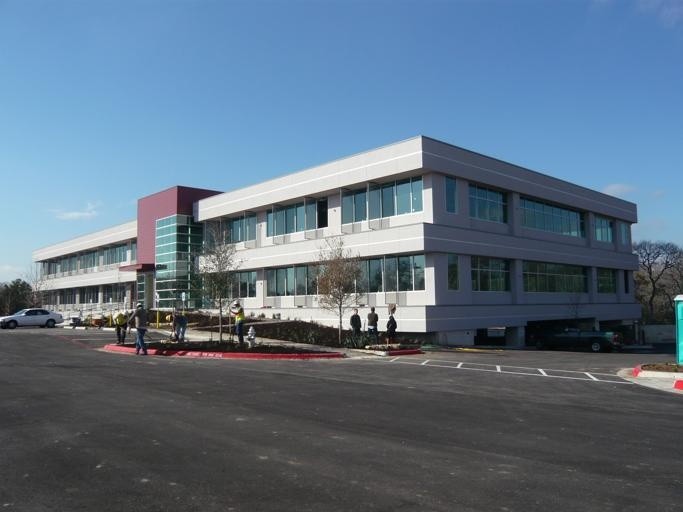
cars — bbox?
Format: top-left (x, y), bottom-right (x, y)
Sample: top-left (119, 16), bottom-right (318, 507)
top-left (0, 307), bottom-right (64, 330)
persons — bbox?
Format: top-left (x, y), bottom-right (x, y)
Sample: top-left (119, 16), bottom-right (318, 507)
top-left (113, 312), bottom-right (127, 344)
top-left (166, 314), bottom-right (187, 344)
top-left (367, 307), bottom-right (378, 344)
top-left (127, 302), bottom-right (148, 355)
top-left (350, 308), bottom-right (361, 338)
top-left (386, 316), bottom-right (397, 347)
top-left (230, 300), bottom-right (245, 345)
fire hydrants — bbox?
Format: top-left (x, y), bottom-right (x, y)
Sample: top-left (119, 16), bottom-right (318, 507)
top-left (245, 325), bottom-right (257, 349)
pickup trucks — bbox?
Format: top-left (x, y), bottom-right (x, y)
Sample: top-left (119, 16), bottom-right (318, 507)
top-left (533, 322), bottom-right (628, 354)
